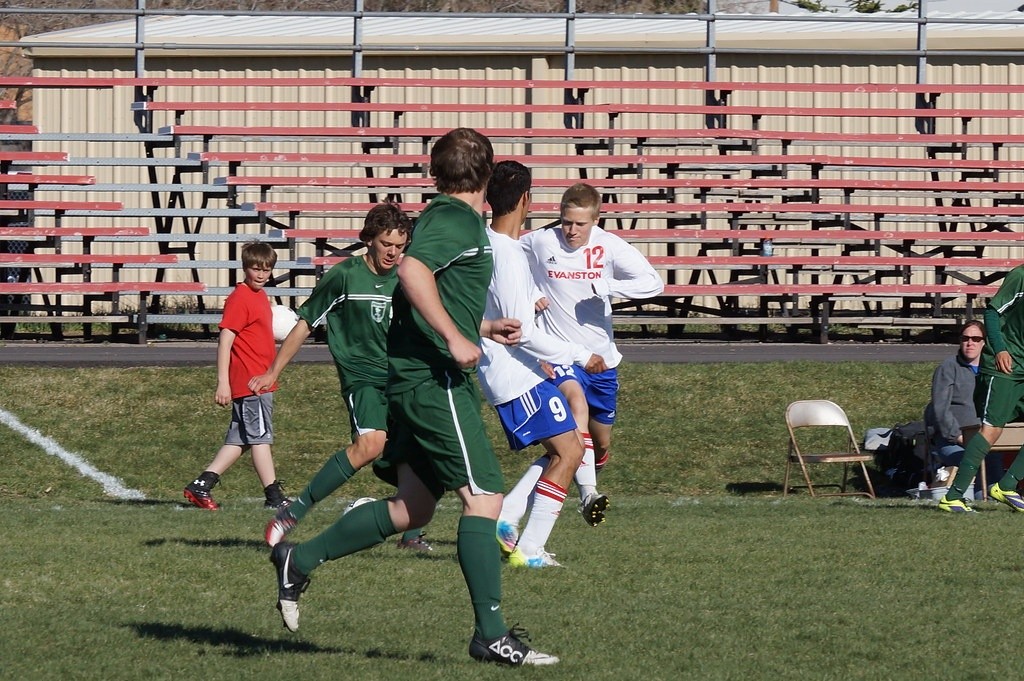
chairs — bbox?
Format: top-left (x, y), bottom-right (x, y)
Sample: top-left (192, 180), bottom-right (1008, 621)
top-left (783, 400), bottom-right (877, 499)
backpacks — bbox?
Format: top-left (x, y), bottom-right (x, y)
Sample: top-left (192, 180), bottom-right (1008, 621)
top-left (864, 420), bottom-right (943, 497)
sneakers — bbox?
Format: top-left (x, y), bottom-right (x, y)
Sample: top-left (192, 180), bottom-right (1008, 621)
top-left (263, 496), bottom-right (300, 514)
top-left (396, 531), bottom-right (433, 553)
top-left (184, 477), bottom-right (217, 511)
top-left (576, 492), bottom-right (611, 528)
top-left (990, 483), bottom-right (1024, 512)
top-left (263, 508), bottom-right (296, 548)
top-left (938, 495), bottom-right (979, 516)
top-left (468, 622), bottom-right (560, 668)
top-left (494, 519), bottom-right (563, 568)
top-left (271, 542), bottom-right (313, 633)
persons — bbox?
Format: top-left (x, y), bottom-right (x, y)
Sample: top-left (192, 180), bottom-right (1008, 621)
top-left (520, 184), bottom-right (664, 525)
top-left (475, 161), bottom-right (608, 567)
top-left (184, 240), bottom-right (294, 510)
top-left (270, 127), bottom-right (560, 667)
top-left (932, 321), bottom-right (1005, 501)
top-left (249, 203), bottom-right (443, 557)
top-left (938, 264), bottom-right (1024, 515)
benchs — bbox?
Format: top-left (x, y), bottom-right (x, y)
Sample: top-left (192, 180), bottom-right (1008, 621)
top-left (0, 72), bottom-right (1024, 341)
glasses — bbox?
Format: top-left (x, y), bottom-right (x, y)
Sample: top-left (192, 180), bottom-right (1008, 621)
top-left (960, 334), bottom-right (985, 342)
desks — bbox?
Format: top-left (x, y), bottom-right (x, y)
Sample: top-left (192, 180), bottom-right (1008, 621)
top-left (959, 423), bottom-right (1024, 502)
